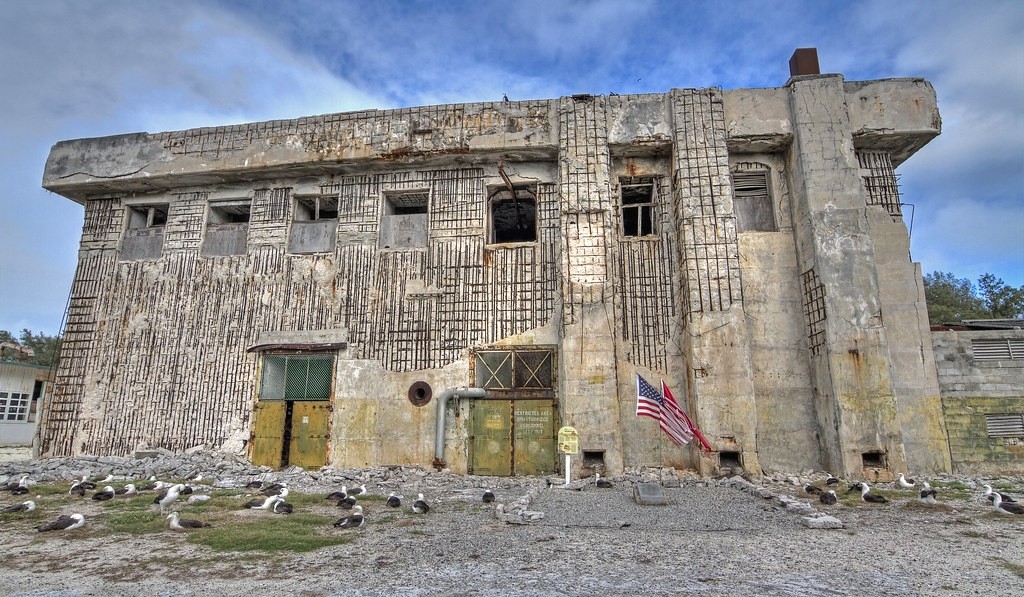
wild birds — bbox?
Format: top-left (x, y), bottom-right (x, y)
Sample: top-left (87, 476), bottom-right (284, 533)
top-left (241, 480), bottom-right (293, 513)
top-left (482, 489), bottom-right (495, 503)
top-left (1, 476), bottom-right (210, 532)
top-left (805, 469), bottom-right (1024, 515)
top-left (386, 496), bottom-right (400, 506)
top-left (595, 472), bottom-right (612, 488)
top-left (412, 492), bottom-right (430, 514)
top-left (325, 480), bottom-right (366, 528)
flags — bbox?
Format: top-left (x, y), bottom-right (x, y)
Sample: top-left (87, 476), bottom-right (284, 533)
top-left (662, 381), bottom-right (712, 451)
top-left (637, 376), bottom-right (694, 450)
